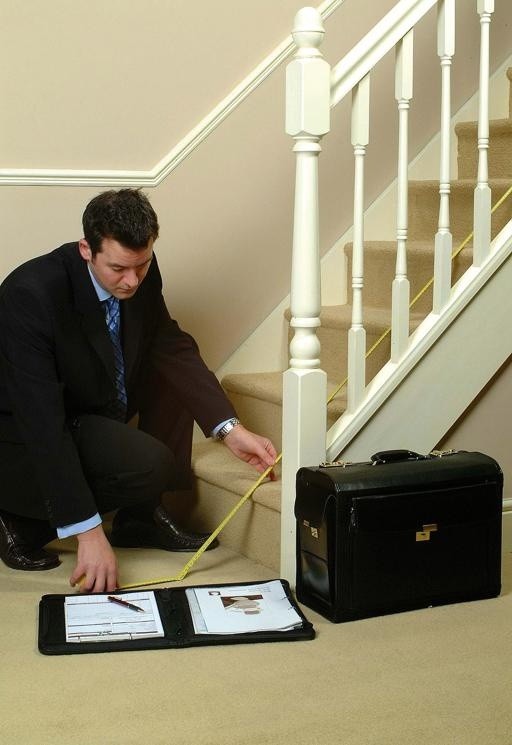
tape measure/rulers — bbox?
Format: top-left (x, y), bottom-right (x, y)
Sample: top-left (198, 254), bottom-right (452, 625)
top-left (74, 188), bottom-right (512, 593)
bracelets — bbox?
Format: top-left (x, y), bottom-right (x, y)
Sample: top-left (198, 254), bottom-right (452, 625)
top-left (217, 418), bottom-right (241, 442)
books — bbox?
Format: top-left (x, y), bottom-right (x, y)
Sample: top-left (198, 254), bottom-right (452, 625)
top-left (63, 591), bottom-right (166, 645)
top-left (185, 581), bottom-right (305, 637)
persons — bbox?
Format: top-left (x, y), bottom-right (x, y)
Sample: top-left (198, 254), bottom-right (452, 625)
top-left (1, 188), bottom-right (280, 593)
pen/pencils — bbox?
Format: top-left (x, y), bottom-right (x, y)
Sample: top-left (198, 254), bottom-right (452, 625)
top-left (107, 596), bottom-right (144, 612)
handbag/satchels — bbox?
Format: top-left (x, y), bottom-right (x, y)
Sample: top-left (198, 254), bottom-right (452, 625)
top-left (293, 449), bottom-right (505, 623)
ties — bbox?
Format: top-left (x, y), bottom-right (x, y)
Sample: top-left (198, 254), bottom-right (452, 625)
top-left (105, 296), bottom-right (128, 422)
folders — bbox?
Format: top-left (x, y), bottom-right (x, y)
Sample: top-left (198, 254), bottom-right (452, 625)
top-left (38, 579), bottom-right (316, 656)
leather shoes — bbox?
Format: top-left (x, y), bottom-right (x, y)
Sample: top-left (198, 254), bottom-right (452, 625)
top-left (1, 514), bottom-right (60, 571)
top-left (111, 504), bottom-right (219, 553)
